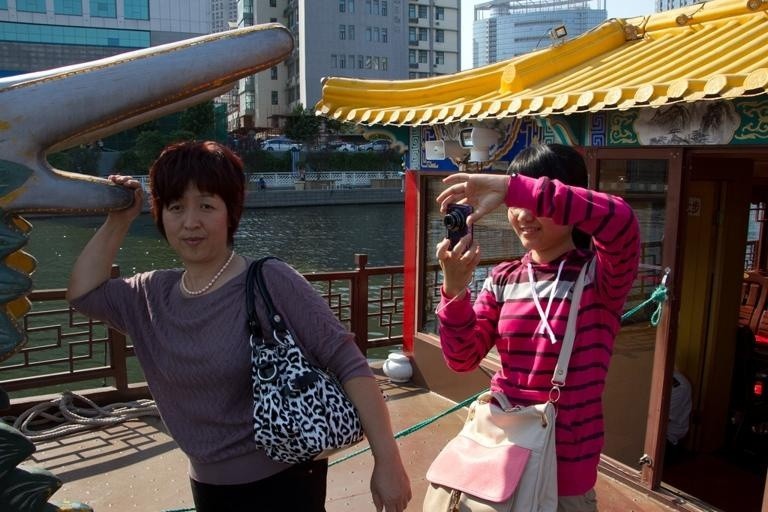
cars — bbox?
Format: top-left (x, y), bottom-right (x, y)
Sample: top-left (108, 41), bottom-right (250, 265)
top-left (326, 138), bottom-right (392, 152)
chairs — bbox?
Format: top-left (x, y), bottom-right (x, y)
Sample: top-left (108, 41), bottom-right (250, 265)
top-left (738, 271), bottom-right (768, 369)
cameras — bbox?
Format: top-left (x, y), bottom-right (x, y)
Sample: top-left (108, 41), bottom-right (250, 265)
top-left (443, 202), bottom-right (474, 252)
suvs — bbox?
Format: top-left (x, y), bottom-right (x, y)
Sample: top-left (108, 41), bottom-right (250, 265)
top-left (259, 138), bottom-right (303, 153)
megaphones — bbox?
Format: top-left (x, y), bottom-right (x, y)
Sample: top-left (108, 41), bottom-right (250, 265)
top-left (425, 139), bottom-right (462, 161)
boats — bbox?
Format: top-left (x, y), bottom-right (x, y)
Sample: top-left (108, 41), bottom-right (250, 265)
top-left (0, 2), bottom-right (768, 511)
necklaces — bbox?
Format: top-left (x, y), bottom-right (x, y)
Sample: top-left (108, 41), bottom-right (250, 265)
top-left (181, 249), bottom-right (235, 296)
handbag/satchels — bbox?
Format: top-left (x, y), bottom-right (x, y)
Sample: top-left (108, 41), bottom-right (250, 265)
top-left (243, 256), bottom-right (365, 465)
top-left (420, 388), bottom-right (561, 511)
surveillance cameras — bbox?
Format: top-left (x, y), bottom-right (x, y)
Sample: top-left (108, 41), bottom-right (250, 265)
top-left (458, 126), bottom-right (499, 149)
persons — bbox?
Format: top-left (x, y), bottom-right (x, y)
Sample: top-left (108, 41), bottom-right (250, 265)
top-left (256, 175), bottom-right (266, 192)
top-left (435, 142), bottom-right (641, 510)
top-left (299, 166), bottom-right (306, 181)
top-left (66, 139), bottom-right (411, 512)
